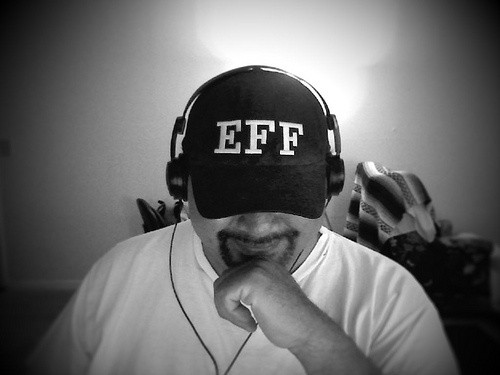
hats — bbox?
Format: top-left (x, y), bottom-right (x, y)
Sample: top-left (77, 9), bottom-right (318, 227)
top-left (182, 67), bottom-right (329, 219)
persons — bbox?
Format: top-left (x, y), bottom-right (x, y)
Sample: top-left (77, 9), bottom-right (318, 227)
top-left (24, 66), bottom-right (459, 375)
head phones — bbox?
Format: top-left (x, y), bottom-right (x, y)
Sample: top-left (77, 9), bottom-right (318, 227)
top-left (166, 64), bottom-right (345, 203)
